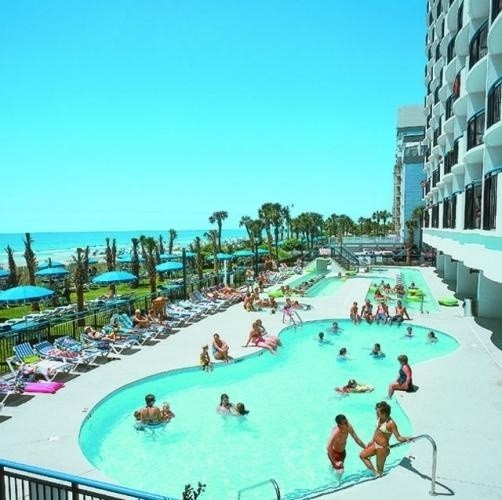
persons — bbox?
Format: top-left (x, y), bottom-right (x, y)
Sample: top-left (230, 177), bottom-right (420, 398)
top-left (242, 319), bottom-right (280, 353)
top-left (133, 296), bottom-right (169, 328)
top-left (294, 259), bottom-right (303, 271)
top-left (373, 343), bottom-right (383, 356)
top-left (200, 345), bottom-right (210, 369)
top-left (134, 394), bottom-right (172, 424)
top-left (344, 379), bottom-right (369, 392)
top-left (220, 394), bottom-right (247, 416)
top-left (389, 354), bottom-right (418, 396)
top-left (349, 280), bottom-right (416, 327)
top-left (83, 325), bottom-right (115, 341)
top-left (212, 333), bottom-right (232, 361)
top-left (340, 348), bottom-right (346, 355)
top-left (319, 332), bottom-right (324, 340)
top-left (203, 282), bottom-right (310, 321)
top-left (326, 413), bottom-right (366, 485)
top-left (428, 331), bottom-right (437, 342)
top-left (359, 401), bottom-right (414, 478)
top-left (245, 259), bottom-right (278, 292)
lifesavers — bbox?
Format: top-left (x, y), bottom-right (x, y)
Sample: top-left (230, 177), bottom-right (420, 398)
top-left (406, 287), bottom-right (424, 301)
top-left (438, 297), bottom-right (459, 307)
top-left (369, 285), bottom-right (380, 292)
top-left (267, 287), bottom-right (306, 298)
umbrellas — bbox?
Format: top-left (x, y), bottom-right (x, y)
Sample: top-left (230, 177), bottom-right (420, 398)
top-left (1, 246), bottom-right (269, 323)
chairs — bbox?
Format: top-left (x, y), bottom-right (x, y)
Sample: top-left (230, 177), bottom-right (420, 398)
top-left (0, 283), bottom-right (243, 406)
